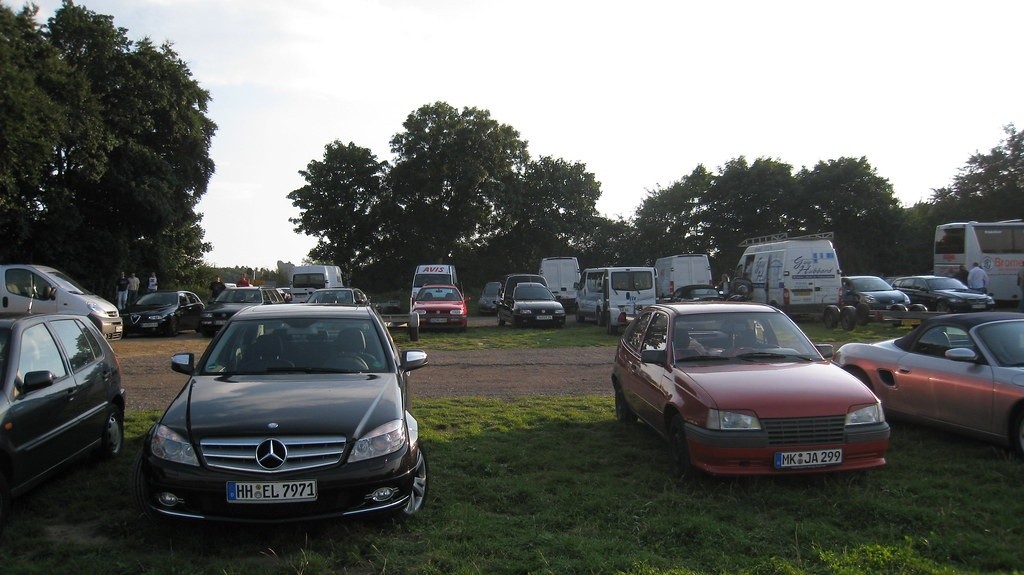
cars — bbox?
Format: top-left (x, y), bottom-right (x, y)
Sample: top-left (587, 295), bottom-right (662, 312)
top-left (123, 285), bottom-right (286, 337)
top-left (478, 281), bottom-right (503, 316)
top-left (306, 287), bottom-right (367, 303)
top-left (134, 302), bottom-right (428, 537)
top-left (412, 284), bottom-right (471, 334)
top-left (831, 311), bottom-right (1024, 452)
top-left (841, 275), bottom-right (911, 311)
top-left (659, 284), bottom-right (728, 301)
top-left (891, 275), bottom-right (995, 315)
top-left (0, 314), bottom-right (126, 504)
top-left (609, 301), bottom-right (891, 477)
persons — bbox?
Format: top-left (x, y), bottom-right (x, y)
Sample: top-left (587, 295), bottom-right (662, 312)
top-left (1017, 260), bottom-right (1024, 313)
top-left (712, 272), bottom-right (754, 300)
top-left (237, 273), bottom-right (254, 298)
top-left (115, 271), bottom-right (140, 310)
top-left (146, 271), bottom-right (158, 293)
top-left (955, 262), bottom-right (989, 294)
top-left (208, 275), bottom-right (226, 302)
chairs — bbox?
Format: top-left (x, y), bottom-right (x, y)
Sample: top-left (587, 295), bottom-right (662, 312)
top-left (423, 292), bottom-right (434, 300)
top-left (667, 329), bottom-right (700, 360)
top-left (321, 294), bottom-right (338, 303)
top-left (445, 293), bottom-right (457, 301)
top-left (321, 328), bottom-right (378, 371)
top-left (26, 275), bottom-right (38, 298)
top-left (239, 336), bottom-right (292, 374)
top-left (233, 293), bottom-right (248, 301)
top-left (931, 330), bottom-right (952, 355)
top-left (252, 293), bottom-right (261, 301)
top-left (8, 282), bottom-right (21, 294)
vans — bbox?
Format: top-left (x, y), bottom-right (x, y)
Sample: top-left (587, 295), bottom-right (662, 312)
top-left (572, 267), bottom-right (656, 326)
top-left (412, 264), bottom-right (458, 299)
top-left (0, 264), bottom-right (123, 344)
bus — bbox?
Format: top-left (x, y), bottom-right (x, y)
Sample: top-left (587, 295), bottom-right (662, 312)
top-left (933, 219), bottom-right (1024, 301)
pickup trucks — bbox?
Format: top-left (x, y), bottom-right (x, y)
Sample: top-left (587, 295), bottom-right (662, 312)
top-left (492, 273), bottom-right (567, 328)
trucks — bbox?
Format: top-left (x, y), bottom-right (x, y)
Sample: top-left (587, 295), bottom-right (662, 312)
top-left (655, 253), bottom-right (713, 296)
top-left (734, 240), bottom-right (841, 315)
top-left (289, 264), bottom-right (344, 303)
top-left (539, 257), bottom-right (582, 310)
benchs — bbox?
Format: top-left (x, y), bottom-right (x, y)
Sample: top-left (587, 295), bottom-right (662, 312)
top-left (689, 329), bottom-right (730, 348)
